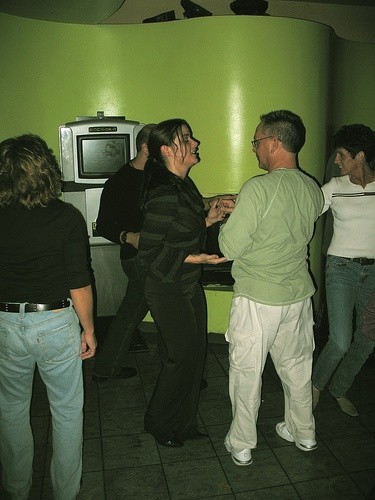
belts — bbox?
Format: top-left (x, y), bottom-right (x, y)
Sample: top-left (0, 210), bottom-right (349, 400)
top-left (0, 300), bottom-right (71, 313)
top-left (344, 256), bottom-right (375, 266)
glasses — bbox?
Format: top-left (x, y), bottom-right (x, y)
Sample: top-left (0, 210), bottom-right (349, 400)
top-left (251, 135), bottom-right (281, 147)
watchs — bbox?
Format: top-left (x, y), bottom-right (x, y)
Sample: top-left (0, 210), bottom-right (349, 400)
top-left (121, 231), bottom-right (130, 245)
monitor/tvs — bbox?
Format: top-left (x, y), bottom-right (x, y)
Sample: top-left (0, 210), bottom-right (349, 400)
top-left (77, 134), bottom-right (130, 178)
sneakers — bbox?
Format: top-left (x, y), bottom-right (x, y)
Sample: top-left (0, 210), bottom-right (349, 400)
top-left (230, 447), bottom-right (254, 466)
top-left (275, 421), bottom-right (319, 452)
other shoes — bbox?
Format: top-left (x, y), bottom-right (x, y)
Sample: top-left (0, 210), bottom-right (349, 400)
top-left (91, 367), bottom-right (138, 382)
top-left (312, 384), bottom-right (321, 413)
top-left (181, 428), bottom-right (209, 441)
top-left (148, 429), bottom-right (185, 448)
top-left (335, 396), bottom-right (360, 417)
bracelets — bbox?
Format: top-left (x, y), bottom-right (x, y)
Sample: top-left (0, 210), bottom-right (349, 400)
top-left (207, 200), bottom-right (211, 208)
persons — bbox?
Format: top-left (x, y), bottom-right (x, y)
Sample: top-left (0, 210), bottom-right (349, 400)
top-left (91, 124), bottom-right (209, 390)
top-left (218, 110), bottom-right (325, 466)
top-left (0, 132), bottom-right (98, 500)
top-left (311, 123), bottom-right (375, 417)
top-left (137, 118), bottom-right (229, 448)
